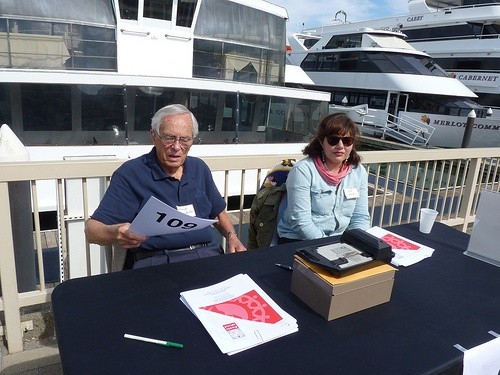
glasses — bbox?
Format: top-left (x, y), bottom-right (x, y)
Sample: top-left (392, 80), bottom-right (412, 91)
top-left (156, 132), bottom-right (195, 148)
top-left (326, 136), bottom-right (354, 146)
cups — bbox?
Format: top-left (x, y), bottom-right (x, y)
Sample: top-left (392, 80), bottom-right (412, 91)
top-left (419, 207), bottom-right (439, 235)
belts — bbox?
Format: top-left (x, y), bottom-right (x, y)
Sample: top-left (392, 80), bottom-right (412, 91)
top-left (135, 240), bottom-right (213, 261)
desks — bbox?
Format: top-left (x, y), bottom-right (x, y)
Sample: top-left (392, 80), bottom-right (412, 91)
top-left (52, 221), bottom-right (500, 375)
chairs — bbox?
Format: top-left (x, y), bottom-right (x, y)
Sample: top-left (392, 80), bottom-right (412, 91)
top-left (249, 160), bottom-right (302, 249)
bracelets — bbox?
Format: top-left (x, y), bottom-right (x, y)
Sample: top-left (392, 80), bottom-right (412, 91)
top-left (225, 230), bottom-right (237, 239)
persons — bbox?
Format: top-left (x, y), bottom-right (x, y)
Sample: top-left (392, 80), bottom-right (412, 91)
top-left (275, 112), bottom-right (371, 245)
top-left (84, 104), bottom-right (247, 269)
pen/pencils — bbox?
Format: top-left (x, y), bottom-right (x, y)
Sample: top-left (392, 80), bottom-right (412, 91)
top-left (275, 263), bottom-right (292, 269)
top-left (124, 335), bottom-right (184, 349)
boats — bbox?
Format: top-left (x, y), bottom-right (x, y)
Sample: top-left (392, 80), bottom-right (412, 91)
top-left (224, 0), bottom-right (500, 179)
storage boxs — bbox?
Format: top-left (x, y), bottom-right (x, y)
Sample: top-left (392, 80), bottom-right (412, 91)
top-left (290, 254), bottom-right (396, 321)
